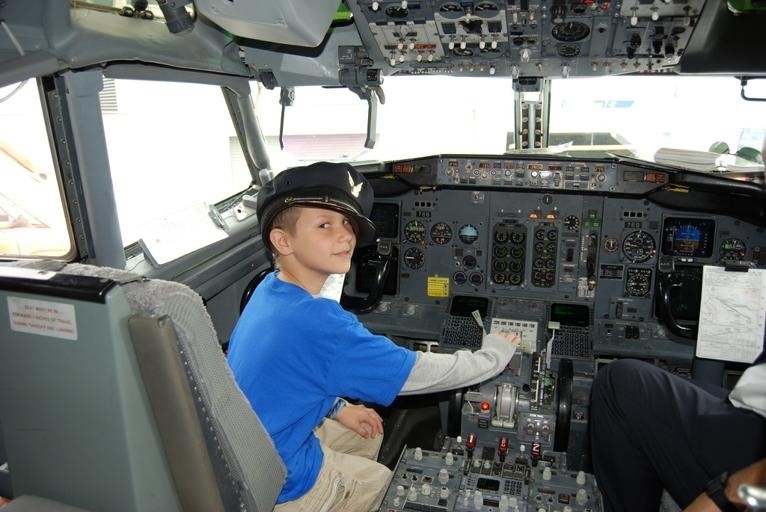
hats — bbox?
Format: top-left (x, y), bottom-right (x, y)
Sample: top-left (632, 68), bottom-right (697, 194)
top-left (256, 160), bottom-right (377, 252)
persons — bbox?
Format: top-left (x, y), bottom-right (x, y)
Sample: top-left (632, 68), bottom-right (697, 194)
top-left (587, 315), bottom-right (766, 512)
top-left (226, 159), bottom-right (525, 512)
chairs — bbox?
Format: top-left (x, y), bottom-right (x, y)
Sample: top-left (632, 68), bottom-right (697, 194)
top-left (0, 257), bottom-right (287, 512)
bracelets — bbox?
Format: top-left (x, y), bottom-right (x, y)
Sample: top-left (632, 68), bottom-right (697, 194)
top-left (703, 472), bottom-right (737, 512)
